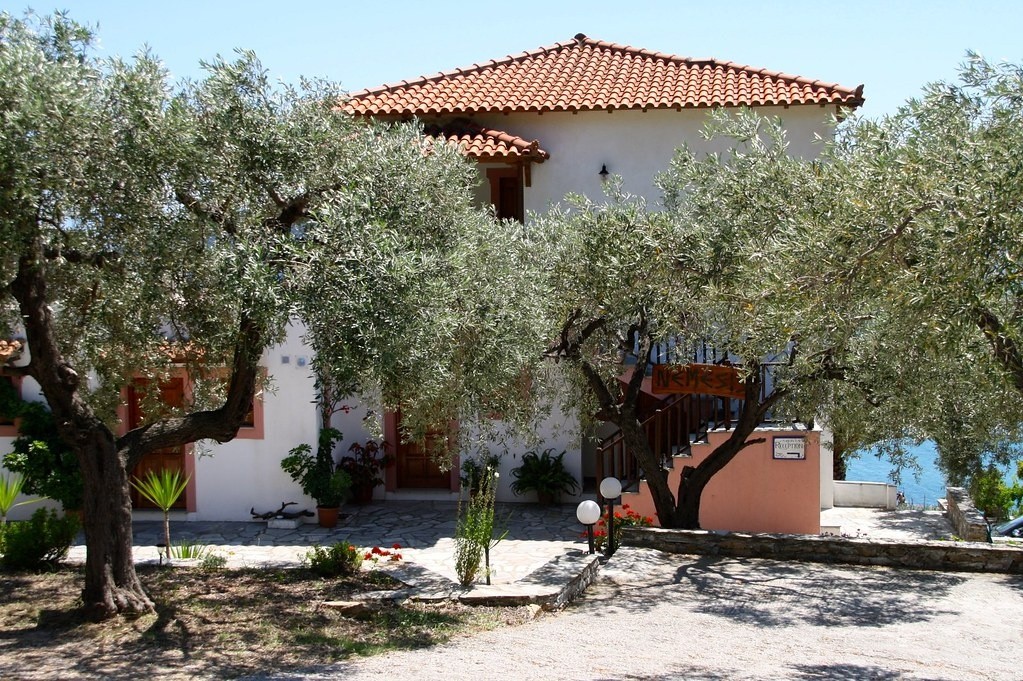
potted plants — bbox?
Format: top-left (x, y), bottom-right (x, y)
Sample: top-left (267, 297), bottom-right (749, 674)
top-left (457, 453), bottom-right (502, 495)
top-left (281, 425), bottom-right (354, 527)
top-left (249, 502), bottom-right (316, 529)
top-left (336, 439), bottom-right (395, 506)
top-left (509, 448), bottom-right (580, 505)
top-left (1, 396), bottom-right (88, 526)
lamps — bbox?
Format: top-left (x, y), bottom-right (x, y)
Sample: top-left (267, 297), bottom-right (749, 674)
top-left (598, 163), bottom-right (610, 180)
top-left (600, 477), bottom-right (623, 500)
top-left (576, 499), bottom-right (601, 526)
